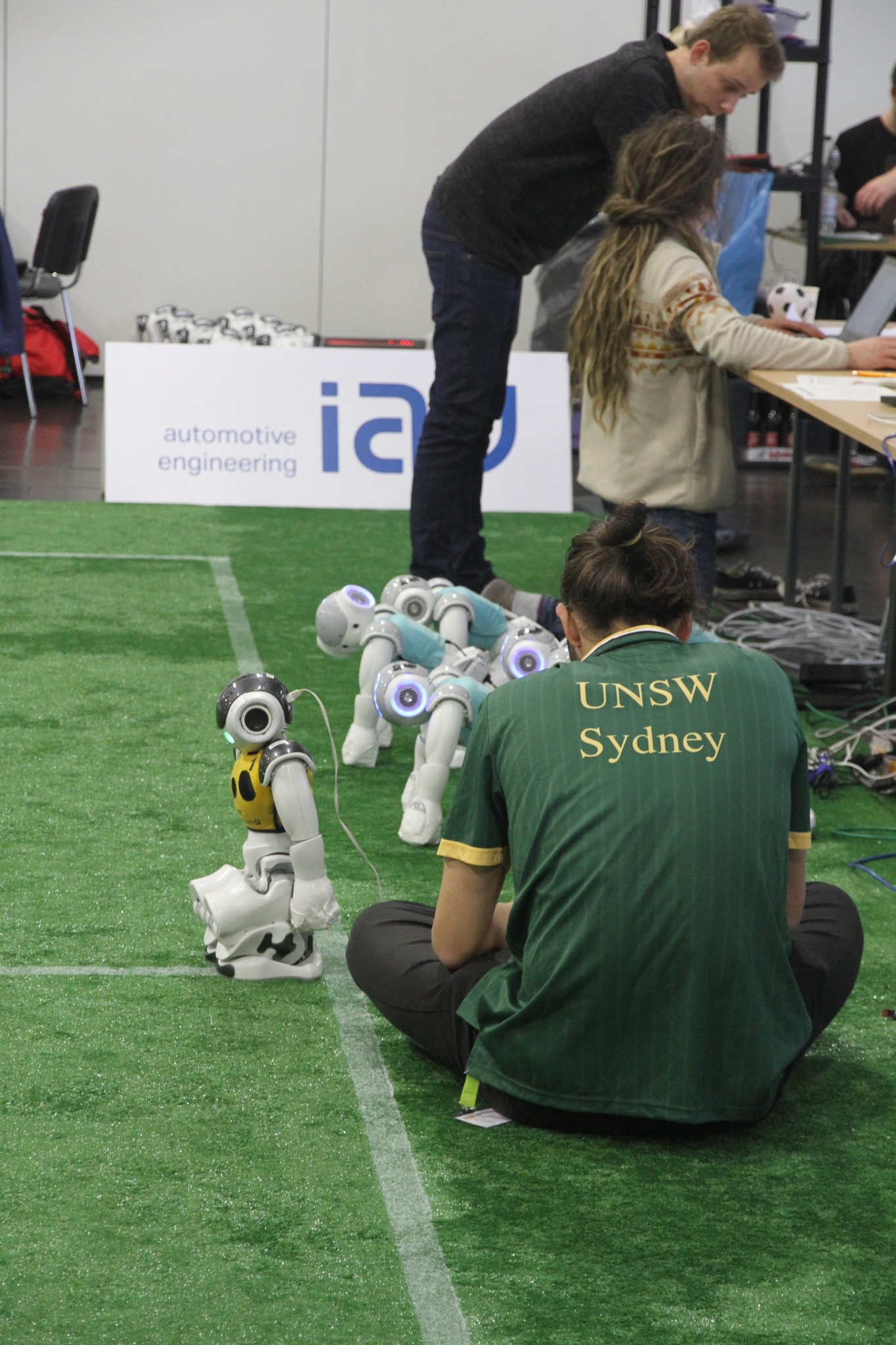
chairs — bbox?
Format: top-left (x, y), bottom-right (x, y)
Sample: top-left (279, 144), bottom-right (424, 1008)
top-left (14, 184), bottom-right (102, 421)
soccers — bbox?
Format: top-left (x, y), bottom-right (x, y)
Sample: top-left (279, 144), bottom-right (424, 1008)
top-left (766, 282), bottom-right (809, 320)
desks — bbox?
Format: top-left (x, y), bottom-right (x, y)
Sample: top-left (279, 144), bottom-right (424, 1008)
top-left (730, 318), bottom-right (896, 699)
top-left (766, 223), bottom-right (896, 319)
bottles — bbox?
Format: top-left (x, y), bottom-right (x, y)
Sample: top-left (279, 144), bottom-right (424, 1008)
top-left (745, 405), bottom-right (761, 449)
top-left (817, 134), bottom-right (841, 236)
top-left (786, 405), bottom-right (795, 448)
top-left (762, 403), bottom-right (782, 447)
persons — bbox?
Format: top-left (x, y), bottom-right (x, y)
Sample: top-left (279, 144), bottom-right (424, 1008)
top-left (347, 496), bottom-right (864, 1145)
top-left (821, 61), bottom-right (896, 235)
top-left (481, 108), bottom-right (896, 644)
top-left (191, 574), bottom-right (571, 981)
top-left (408, 0), bottom-right (784, 600)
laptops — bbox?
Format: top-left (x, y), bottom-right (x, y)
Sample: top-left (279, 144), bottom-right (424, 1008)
top-left (837, 256), bottom-right (896, 343)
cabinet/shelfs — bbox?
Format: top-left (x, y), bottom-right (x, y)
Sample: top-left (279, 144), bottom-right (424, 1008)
top-left (645, 0), bottom-right (832, 473)
